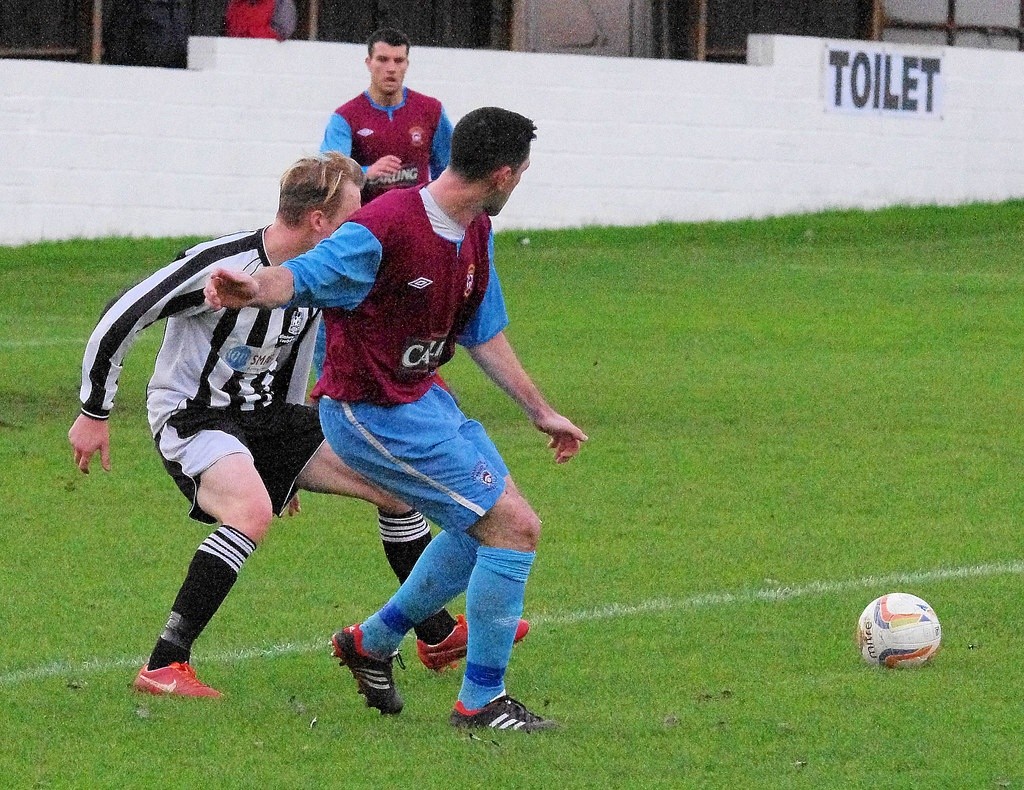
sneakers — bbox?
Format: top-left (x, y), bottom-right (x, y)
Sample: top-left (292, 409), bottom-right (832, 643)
top-left (416, 614), bottom-right (529, 672)
top-left (329, 624), bottom-right (404, 713)
top-left (449, 697), bottom-right (558, 733)
top-left (134, 661), bottom-right (222, 696)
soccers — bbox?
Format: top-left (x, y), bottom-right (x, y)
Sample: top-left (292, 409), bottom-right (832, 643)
top-left (858, 593), bottom-right (942, 667)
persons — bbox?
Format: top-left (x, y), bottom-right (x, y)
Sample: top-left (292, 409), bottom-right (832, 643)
top-left (316, 26), bottom-right (456, 381)
top-left (65, 152), bottom-right (530, 700)
top-left (203, 106), bottom-right (589, 735)
